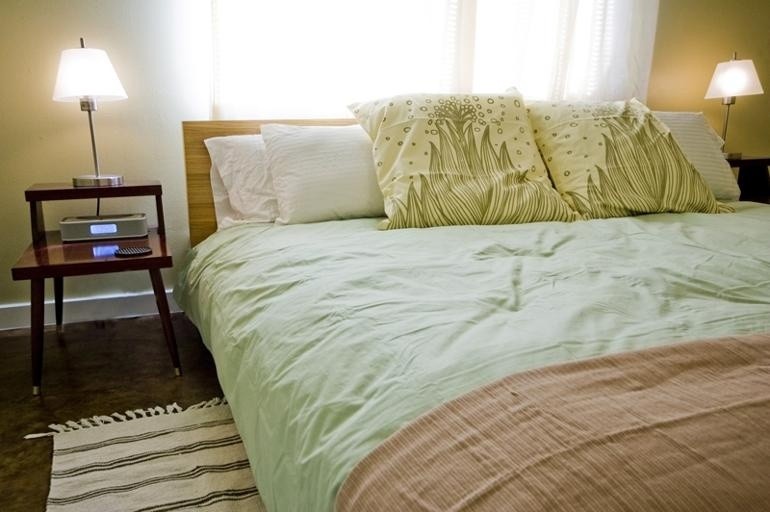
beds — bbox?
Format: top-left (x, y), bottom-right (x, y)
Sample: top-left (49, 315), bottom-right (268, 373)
top-left (181, 118), bottom-right (768, 512)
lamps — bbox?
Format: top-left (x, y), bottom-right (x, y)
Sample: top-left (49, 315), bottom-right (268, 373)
top-left (702, 51), bottom-right (765, 157)
top-left (51, 35), bottom-right (131, 189)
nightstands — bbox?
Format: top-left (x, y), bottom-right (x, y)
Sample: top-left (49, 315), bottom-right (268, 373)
top-left (11, 180), bottom-right (185, 397)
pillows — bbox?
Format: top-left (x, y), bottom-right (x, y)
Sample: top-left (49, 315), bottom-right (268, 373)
top-left (522, 97), bottom-right (741, 221)
top-left (203, 122), bottom-right (386, 230)
top-left (347, 86), bottom-right (580, 232)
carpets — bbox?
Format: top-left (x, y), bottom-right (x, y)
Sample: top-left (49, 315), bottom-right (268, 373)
top-left (24, 394), bottom-right (267, 511)
